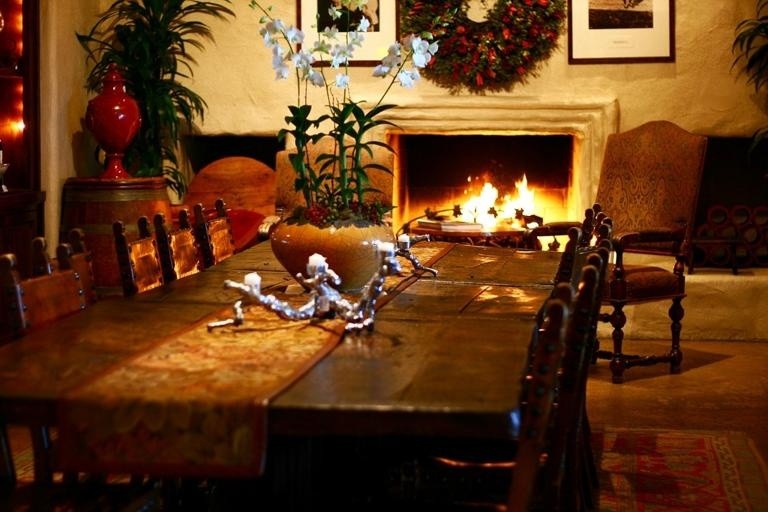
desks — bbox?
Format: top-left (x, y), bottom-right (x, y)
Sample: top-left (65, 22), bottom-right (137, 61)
top-left (59, 175), bottom-right (171, 299)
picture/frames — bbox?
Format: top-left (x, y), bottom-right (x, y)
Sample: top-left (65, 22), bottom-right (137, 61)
top-left (567, 0), bottom-right (677, 65)
top-left (295, 0), bottom-right (400, 68)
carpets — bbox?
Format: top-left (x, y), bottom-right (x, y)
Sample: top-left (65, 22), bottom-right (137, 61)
top-left (593, 264), bottom-right (767, 341)
top-left (432, 424), bottom-right (768, 511)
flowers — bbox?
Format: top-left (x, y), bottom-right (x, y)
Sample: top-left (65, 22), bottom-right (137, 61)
top-left (393, 0), bottom-right (568, 95)
top-left (242, 0), bottom-right (458, 227)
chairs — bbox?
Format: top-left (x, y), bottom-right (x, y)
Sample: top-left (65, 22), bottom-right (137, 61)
top-left (588, 120), bottom-right (708, 384)
top-left (256, 133), bottom-right (394, 242)
top-left (0, 197), bottom-right (617, 512)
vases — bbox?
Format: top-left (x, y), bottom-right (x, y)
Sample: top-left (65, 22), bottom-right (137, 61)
top-left (81, 64), bottom-right (141, 179)
